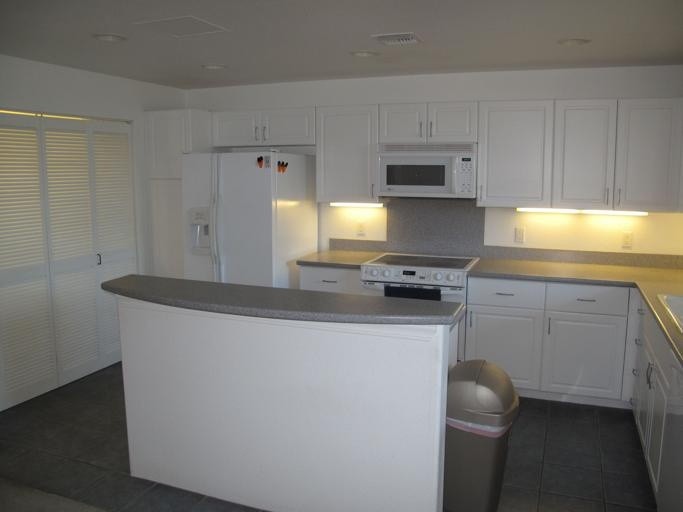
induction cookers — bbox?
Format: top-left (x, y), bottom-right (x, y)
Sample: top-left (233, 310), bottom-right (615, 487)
top-left (361, 252), bottom-right (481, 288)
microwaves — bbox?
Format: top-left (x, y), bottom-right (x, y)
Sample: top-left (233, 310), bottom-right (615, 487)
top-left (377, 150), bottom-right (477, 199)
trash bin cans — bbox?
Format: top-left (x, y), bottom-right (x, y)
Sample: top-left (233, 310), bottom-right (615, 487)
top-left (445, 360), bottom-right (521, 512)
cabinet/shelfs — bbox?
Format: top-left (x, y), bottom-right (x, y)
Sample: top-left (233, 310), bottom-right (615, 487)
top-left (298, 265), bottom-right (385, 298)
top-left (630, 294), bottom-right (674, 511)
top-left (553, 98), bottom-right (683, 215)
top-left (379, 101), bottom-right (478, 143)
top-left (212, 106), bottom-right (316, 148)
top-left (462, 276), bottom-right (630, 412)
top-left (131, 110), bottom-right (212, 180)
top-left (475, 100), bottom-right (554, 209)
top-left (316, 104), bottom-right (379, 203)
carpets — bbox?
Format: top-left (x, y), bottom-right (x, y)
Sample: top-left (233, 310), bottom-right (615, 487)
top-left (0, 359), bottom-right (662, 511)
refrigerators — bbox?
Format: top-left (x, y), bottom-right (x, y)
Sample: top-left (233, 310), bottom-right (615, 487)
top-left (180, 153), bottom-right (319, 289)
